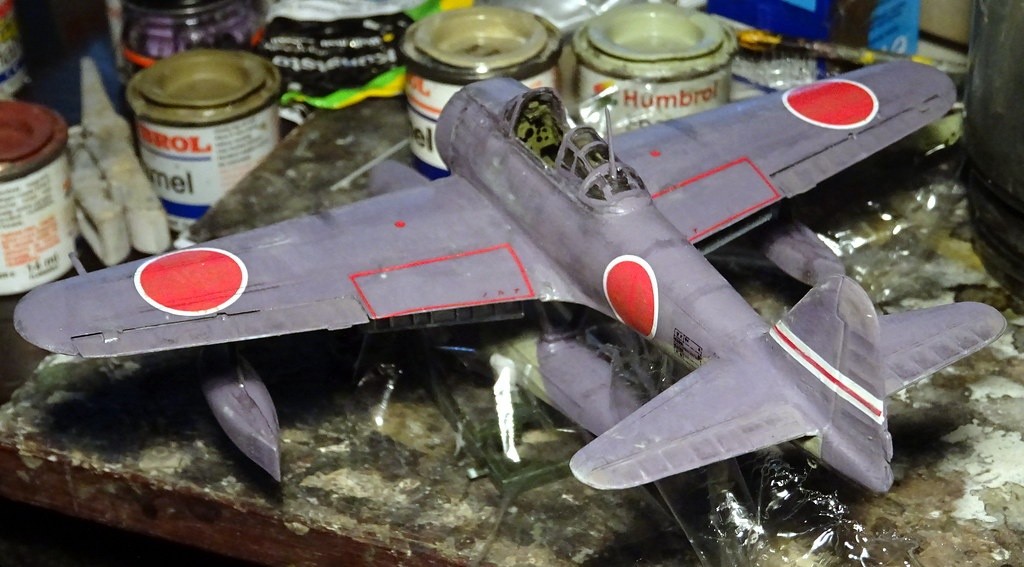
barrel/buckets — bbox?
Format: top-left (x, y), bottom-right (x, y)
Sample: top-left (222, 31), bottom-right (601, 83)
top-left (398, 9), bottom-right (563, 180)
top-left (125, 48), bottom-right (282, 231)
top-left (121, 0), bottom-right (259, 58)
top-left (0, 0), bottom-right (26, 95)
top-left (572, 3), bottom-right (736, 133)
top-left (0, 100), bottom-right (76, 297)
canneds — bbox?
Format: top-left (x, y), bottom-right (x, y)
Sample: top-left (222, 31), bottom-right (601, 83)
top-left (0, 0), bottom-right (742, 296)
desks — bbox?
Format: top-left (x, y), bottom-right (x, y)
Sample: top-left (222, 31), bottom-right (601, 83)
top-left (0, 58), bottom-right (1024, 567)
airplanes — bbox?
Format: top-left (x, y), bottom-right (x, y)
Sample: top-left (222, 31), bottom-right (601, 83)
top-left (11, 57), bottom-right (1009, 497)
top-left (367, 156), bottom-right (653, 434)
